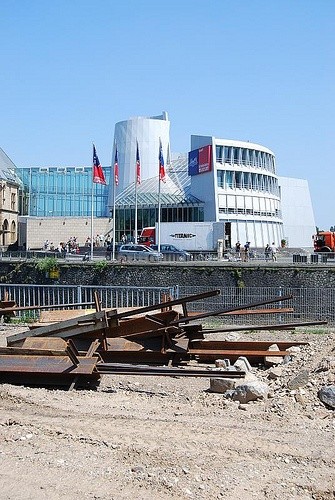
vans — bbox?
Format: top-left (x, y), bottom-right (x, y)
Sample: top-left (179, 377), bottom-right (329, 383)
top-left (117, 244), bottom-right (164, 263)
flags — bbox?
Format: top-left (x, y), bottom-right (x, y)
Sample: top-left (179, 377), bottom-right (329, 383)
top-left (93, 144), bottom-right (166, 185)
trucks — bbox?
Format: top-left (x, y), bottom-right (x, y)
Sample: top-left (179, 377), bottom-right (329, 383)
top-left (137, 221), bottom-right (232, 255)
top-left (312, 231), bottom-right (335, 257)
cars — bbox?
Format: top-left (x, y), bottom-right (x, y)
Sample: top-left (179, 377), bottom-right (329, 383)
top-left (150, 244), bottom-right (188, 262)
top-left (105, 245), bottom-right (121, 261)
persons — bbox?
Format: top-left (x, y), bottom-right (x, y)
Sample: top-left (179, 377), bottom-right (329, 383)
top-left (225, 240), bottom-right (278, 263)
top-left (45, 233), bottom-right (149, 262)
